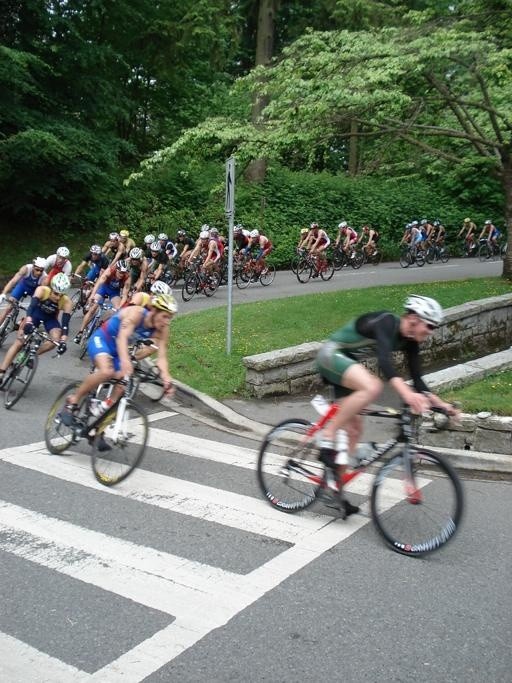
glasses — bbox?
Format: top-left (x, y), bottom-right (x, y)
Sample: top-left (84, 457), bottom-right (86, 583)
top-left (424, 322), bottom-right (437, 328)
top-left (35, 268), bottom-right (43, 272)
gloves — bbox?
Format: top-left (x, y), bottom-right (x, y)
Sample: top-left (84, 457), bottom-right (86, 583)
top-left (23, 323), bottom-right (33, 334)
top-left (58, 342), bottom-right (67, 354)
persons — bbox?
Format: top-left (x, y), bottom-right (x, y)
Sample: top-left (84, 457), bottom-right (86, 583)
top-left (175, 224), bottom-right (272, 285)
top-left (129, 281), bottom-right (173, 361)
top-left (334, 222), bottom-right (357, 260)
top-left (44, 246), bottom-right (72, 277)
top-left (479, 219), bottom-right (501, 259)
top-left (57, 294), bottom-right (178, 450)
top-left (314, 293), bottom-right (461, 517)
top-left (458, 217), bottom-right (478, 256)
top-left (358, 226), bottom-right (379, 256)
top-left (397, 218), bottom-right (446, 261)
top-left (74, 229), bottom-right (177, 340)
top-left (2, 257), bottom-right (50, 322)
top-left (299, 221), bottom-right (330, 277)
top-left (1, 272), bottom-right (71, 379)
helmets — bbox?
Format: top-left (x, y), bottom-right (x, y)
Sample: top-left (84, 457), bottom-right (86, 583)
top-left (90, 224), bottom-right (259, 273)
top-left (301, 222), bottom-right (347, 234)
top-left (152, 294), bottom-right (178, 312)
top-left (403, 293), bottom-right (444, 329)
top-left (363, 226), bottom-right (370, 232)
top-left (51, 272), bottom-right (70, 294)
top-left (34, 256), bottom-right (47, 268)
top-left (405, 219), bottom-right (440, 229)
top-left (56, 246), bottom-right (70, 258)
top-left (464, 218), bottom-right (472, 222)
top-left (485, 219), bottom-right (492, 225)
top-left (150, 280), bottom-right (172, 295)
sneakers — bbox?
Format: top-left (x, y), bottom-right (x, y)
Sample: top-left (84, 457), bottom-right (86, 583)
top-left (62, 395), bottom-right (78, 426)
top-left (350, 252), bottom-right (357, 259)
top-left (315, 428), bottom-right (337, 465)
top-left (74, 331), bottom-right (83, 343)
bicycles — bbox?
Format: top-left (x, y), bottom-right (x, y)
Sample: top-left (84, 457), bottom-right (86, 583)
top-left (453, 237), bottom-right (475, 256)
top-left (398, 246), bottom-right (426, 267)
top-left (2, 330), bottom-right (59, 408)
top-left (476, 237), bottom-right (502, 258)
top-left (181, 267), bottom-right (222, 301)
top-left (334, 247), bottom-right (363, 270)
top-left (426, 244), bottom-right (450, 262)
top-left (0, 227), bottom-right (181, 355)
top-left (46, 360), bottom-right (154, 485)
top-left (354, 247), bottom-right (384, 265)
top-left (288, 250), bottom-right (325, 274)
top-left (256, 391), bottom-right (462, 560)
top-left (297, 259), bottom-right (335, 283)
top-left (235, 261), bottom-right (278, 286)
